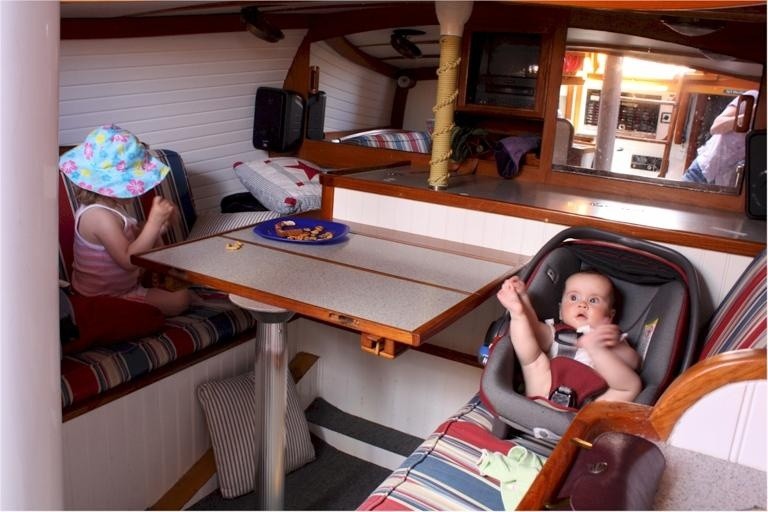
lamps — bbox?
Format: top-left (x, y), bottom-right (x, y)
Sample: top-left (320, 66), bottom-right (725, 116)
top-left (238, 6), bottom-right (286, 43)
top-left (389, 27), bottom-right (425, 60)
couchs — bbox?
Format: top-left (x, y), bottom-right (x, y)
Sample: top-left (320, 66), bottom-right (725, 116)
top-left (58, 144), bottom-right (259, 511)
top-left (353, 243), bottom-right (767, 510)
top-left (321, 124), bottom-right (433, 152)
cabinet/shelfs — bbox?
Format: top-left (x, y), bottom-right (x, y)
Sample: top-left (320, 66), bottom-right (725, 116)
top-left (450, 0), bottom-right (568, 185)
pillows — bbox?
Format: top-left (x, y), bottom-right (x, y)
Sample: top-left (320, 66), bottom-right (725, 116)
top-left (172, 209), bottom-right (280, 313)
top-left (196, 367), bottom-right (317, 498)
top-left (233, 156), bottom-right (330, 213)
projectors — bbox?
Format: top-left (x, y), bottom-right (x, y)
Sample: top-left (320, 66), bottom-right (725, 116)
top-left (391, 35), bottom-right (421, 59)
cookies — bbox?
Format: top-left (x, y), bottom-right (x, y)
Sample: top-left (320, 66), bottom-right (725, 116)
top-left (272, 219), bottom-right (335, 242)
top-left (225, 241), bottom-right (243, 250)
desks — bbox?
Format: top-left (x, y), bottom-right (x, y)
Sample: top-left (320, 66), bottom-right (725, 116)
top-left (129, 215), bottom-right (535, 511)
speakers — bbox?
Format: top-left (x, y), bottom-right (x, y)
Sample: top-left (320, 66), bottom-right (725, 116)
top-left (253, 87), bottom-right (303, 153)
top-left (745, 129), bottom-right (767, 221)
top-left (306, 94), bottom-right (326, 139)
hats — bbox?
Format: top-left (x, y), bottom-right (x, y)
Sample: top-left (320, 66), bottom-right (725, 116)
top-left (59, 124), bottom-right (170, 200)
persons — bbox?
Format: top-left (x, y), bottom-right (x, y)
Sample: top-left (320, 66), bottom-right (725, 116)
top-left (58, 124), bottom-right (200, 314)
top-left (497, 267), bottom-right (641, 415)
top-left (678, 88), bottom-right (759, 187)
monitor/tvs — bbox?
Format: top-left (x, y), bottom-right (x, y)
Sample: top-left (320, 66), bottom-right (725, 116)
top-left (465, 32), bottom-right (543, 111)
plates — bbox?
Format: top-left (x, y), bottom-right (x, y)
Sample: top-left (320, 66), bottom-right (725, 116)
top-left (253, 217), bottom-right (351, 245)
